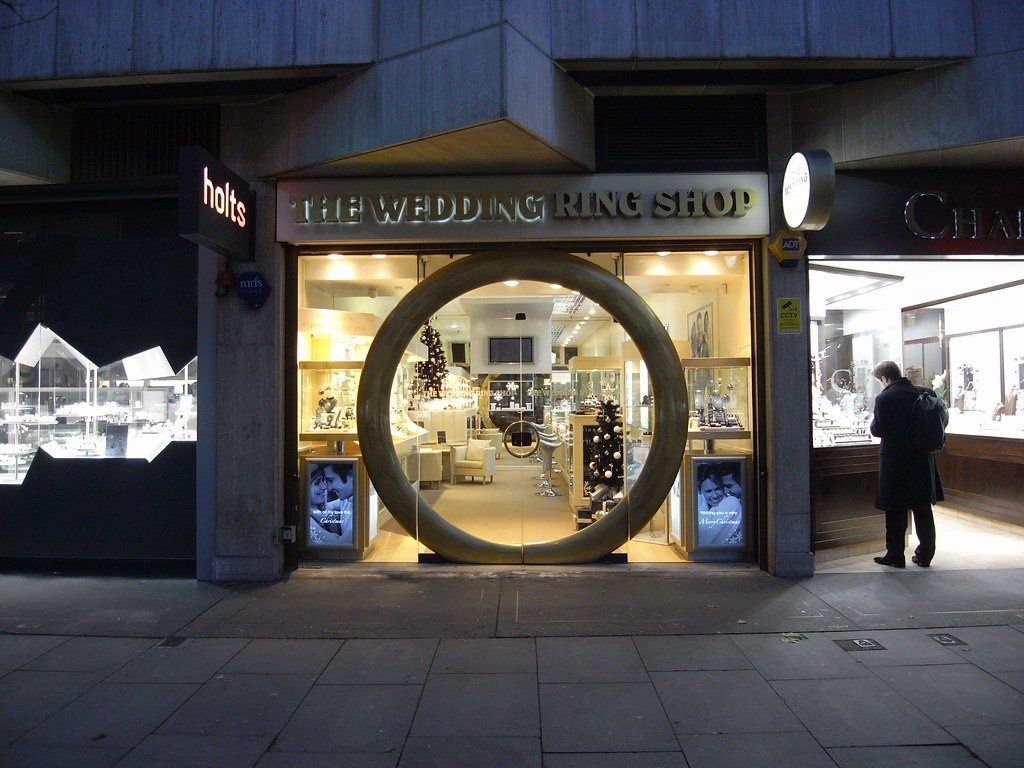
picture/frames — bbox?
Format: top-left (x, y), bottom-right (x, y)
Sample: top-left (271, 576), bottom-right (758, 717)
top-left (687, 302), bottom-right (716, 407)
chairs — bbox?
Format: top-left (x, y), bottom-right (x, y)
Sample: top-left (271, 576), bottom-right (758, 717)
top-left (452, 446), bottom-right (497, 485)
top-left (477, 433), bottom-right (503, 459)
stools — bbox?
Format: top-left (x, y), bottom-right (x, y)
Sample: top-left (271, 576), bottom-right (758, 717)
top-left (408, 450), bottom-right (443, 490)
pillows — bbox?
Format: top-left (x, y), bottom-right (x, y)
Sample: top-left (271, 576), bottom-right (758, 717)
top-left (465, 438), bottom-right (492, 460)
top-left (482, 428), bottom-right (500, 433)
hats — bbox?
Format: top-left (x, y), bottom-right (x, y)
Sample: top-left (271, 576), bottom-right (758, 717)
top-left (310, 464), bottom-right (325, 483)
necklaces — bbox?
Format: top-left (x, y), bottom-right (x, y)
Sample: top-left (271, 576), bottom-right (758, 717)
top-left (1008, 393), bottom-right (1014, 404)
top-left (326, 398), bottom-right (335, 406)
top-left (955, 390), bottom-right (965, 400)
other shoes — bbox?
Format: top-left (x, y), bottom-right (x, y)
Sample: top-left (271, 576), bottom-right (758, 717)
top-left (912, 555), bottom-right (930, 567)
top-left (874, 553), bottom-right (906, 568)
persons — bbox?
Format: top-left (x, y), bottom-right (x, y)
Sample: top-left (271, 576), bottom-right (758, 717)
top-left (869, 361), bottom-right (945, 569)
top-left (697, 461), bottom-right (742, 544)
top-left (309, 464), bottom-right (353, 543)
top-left (691, 311), bottom-right (713, 393)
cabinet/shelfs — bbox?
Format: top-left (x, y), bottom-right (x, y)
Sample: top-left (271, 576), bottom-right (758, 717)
top-left (619, 338), bottom-right (692, 545)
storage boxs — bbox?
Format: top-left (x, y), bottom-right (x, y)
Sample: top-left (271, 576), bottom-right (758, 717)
top-left (297, 360), bottom-right (361, 441)
top-left (568, 355), bottom-right (622, 416)
top-left (678, 357), bottom-right (751, 439)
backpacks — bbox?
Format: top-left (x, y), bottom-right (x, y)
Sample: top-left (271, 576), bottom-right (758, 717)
top-left (907, 383), bottom-right (949, 452)
